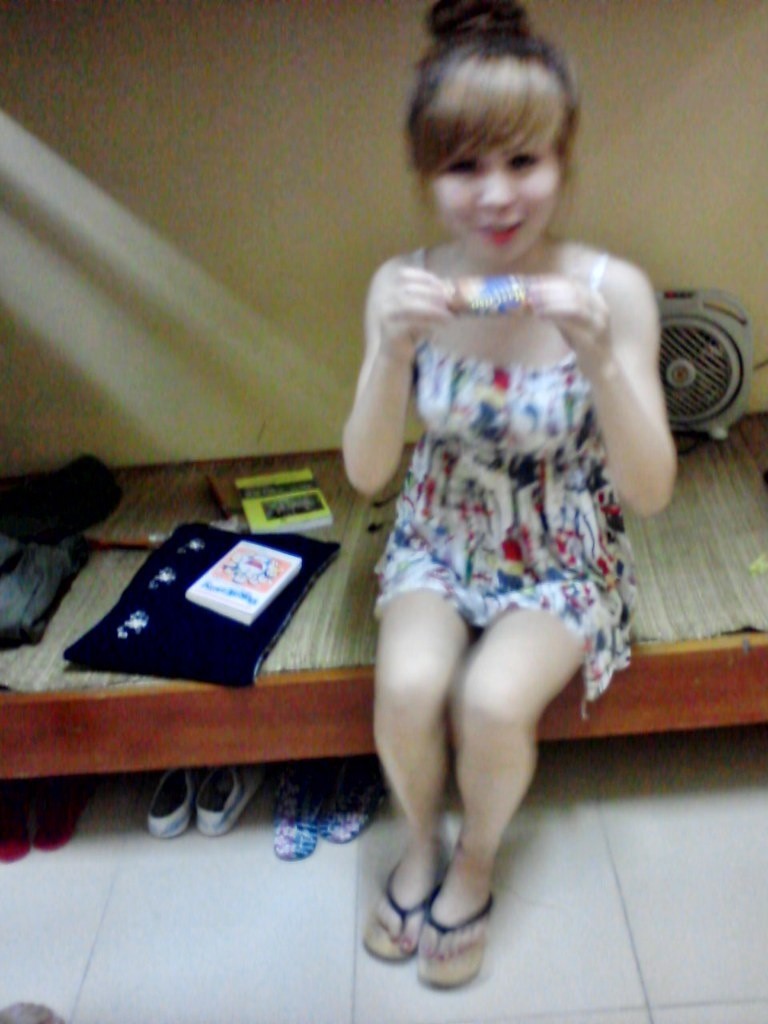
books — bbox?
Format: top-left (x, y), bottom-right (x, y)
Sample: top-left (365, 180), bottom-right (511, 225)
top-left (186, 540), bottom-right (302, 626)
top-left (235, 466), bottom-right (334, 534)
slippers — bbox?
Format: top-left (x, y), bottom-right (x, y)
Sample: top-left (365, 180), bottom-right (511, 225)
top-left (363, 859), bottom-right (439, 961)
top-left (0, 779), bottom-right (30, 861)
top-left (34, 776), bottom-right (89, 849)
top-left (416, 891), bottom-right (497, 987)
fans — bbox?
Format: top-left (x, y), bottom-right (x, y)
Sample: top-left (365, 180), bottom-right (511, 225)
top-left (651, 285), bottom-right (755, 443)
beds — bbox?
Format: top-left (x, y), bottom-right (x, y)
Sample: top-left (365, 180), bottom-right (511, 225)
top-left (0, 408), bottom-right (768, 781)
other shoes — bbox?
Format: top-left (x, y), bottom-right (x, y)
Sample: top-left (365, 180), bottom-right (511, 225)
top-left (323, 767), bottom-right (389, 844)
top-left (271, 759), bottom-right (321, 860)
top-left (147, 767), bottom-right (193, 839)
top-left (193, 762), bottom-right (260, 835)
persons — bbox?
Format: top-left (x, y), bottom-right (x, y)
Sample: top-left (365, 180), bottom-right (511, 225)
top-left (343, 1), bottom-right (680, 991)
